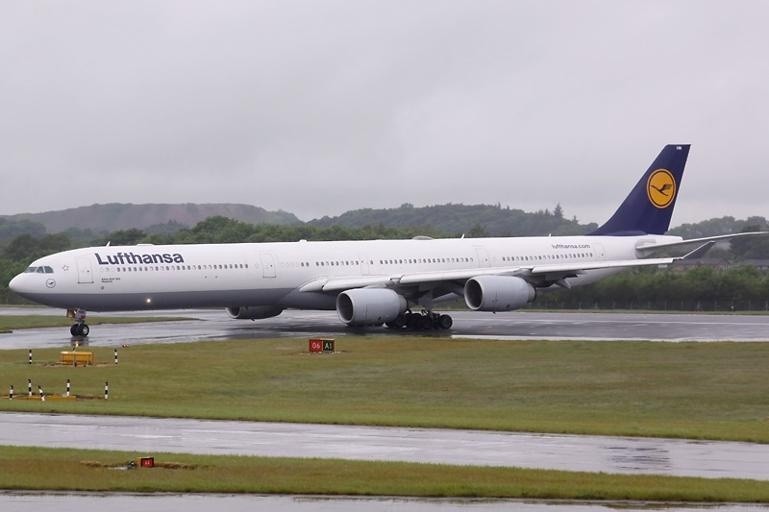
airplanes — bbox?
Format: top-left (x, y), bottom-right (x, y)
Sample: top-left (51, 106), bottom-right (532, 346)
top-left (6, 142), bottom-right (769, 338)
